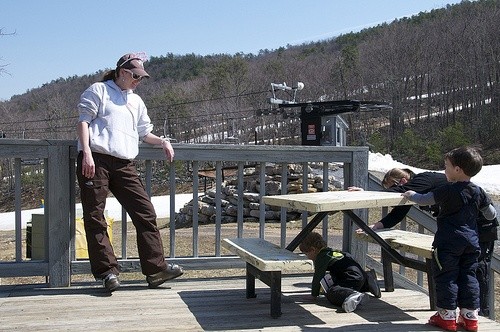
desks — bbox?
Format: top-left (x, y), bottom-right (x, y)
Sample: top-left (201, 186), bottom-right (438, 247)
top-left (262, 190), bottom-right (421, 266)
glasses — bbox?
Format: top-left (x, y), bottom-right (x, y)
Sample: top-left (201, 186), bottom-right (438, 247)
top-left (123, 69), bottom-right (148, 81)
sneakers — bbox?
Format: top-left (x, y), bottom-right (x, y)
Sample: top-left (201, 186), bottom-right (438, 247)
top-left (342, 292), bottom-right (369, 313)
top-left (365, 269), bottom-right (381, 299)
top-left (103, 274), bottom-right (120, 292)
top-left (146, 263), bottom-right (183, 287)
top-left (458, 308), bottom-right (479, 331)
top-left (429, 309), bottom-right (457, 332)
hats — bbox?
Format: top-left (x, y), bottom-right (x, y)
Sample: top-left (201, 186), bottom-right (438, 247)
top-left (116, 53), bottom-right (150, 79)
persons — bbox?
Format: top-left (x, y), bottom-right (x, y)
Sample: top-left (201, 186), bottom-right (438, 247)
top-left (77, 54), bottom-right (183, 294)
top-left (347, 168), bottom-right (499, 316)
top-left (300, 232), bottom-right (381, 313)
top-left (401, 144), bottom-right (497, 332)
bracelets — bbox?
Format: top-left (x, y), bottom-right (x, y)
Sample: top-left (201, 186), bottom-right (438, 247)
top-left (160, 137), bottom-right (171, 146)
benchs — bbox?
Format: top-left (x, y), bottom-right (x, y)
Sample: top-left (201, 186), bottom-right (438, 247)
top-left (221, 236), bottom-right (316, 319)
top-left (355, 225), bottom-right (434, 310)
